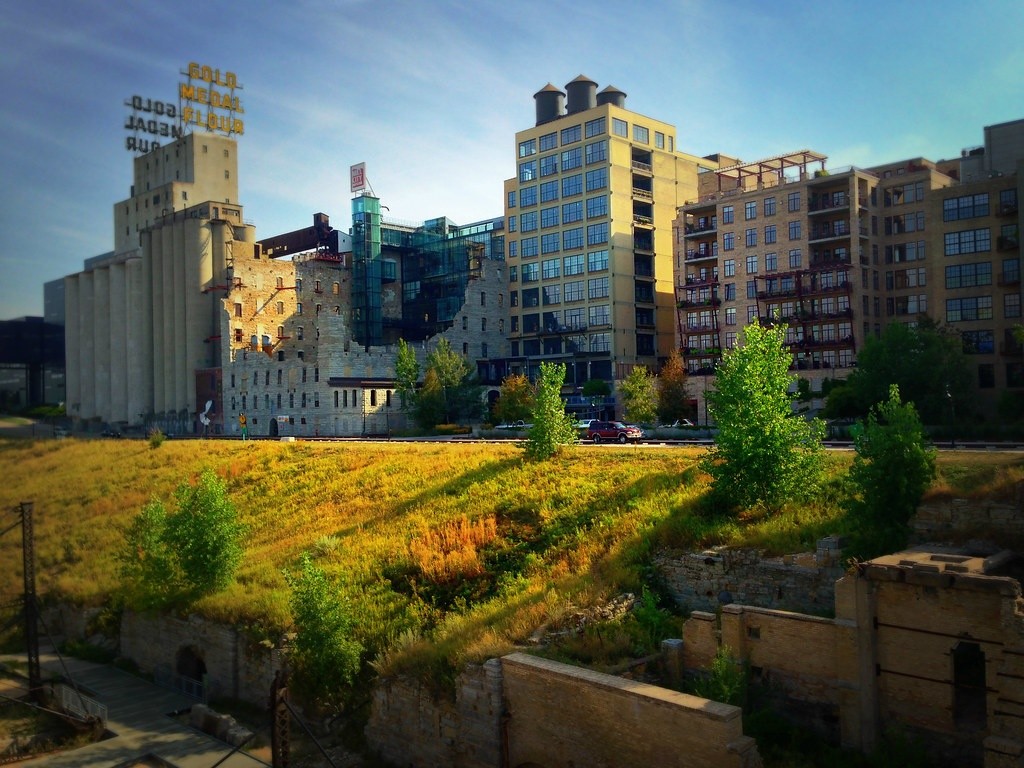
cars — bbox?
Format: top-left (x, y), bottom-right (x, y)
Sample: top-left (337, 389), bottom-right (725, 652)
top-left (54, 425), bottom-right (72, 437)
top-left (100, 429), bottom-right (122, 438)
top-left (572, 418), bottom-right (600, 429)
top-left (658, 419), bottom-right (695, 428)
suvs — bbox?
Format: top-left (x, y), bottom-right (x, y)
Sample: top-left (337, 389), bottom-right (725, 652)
top-left (586, 420), bottom-right (642, 444)
